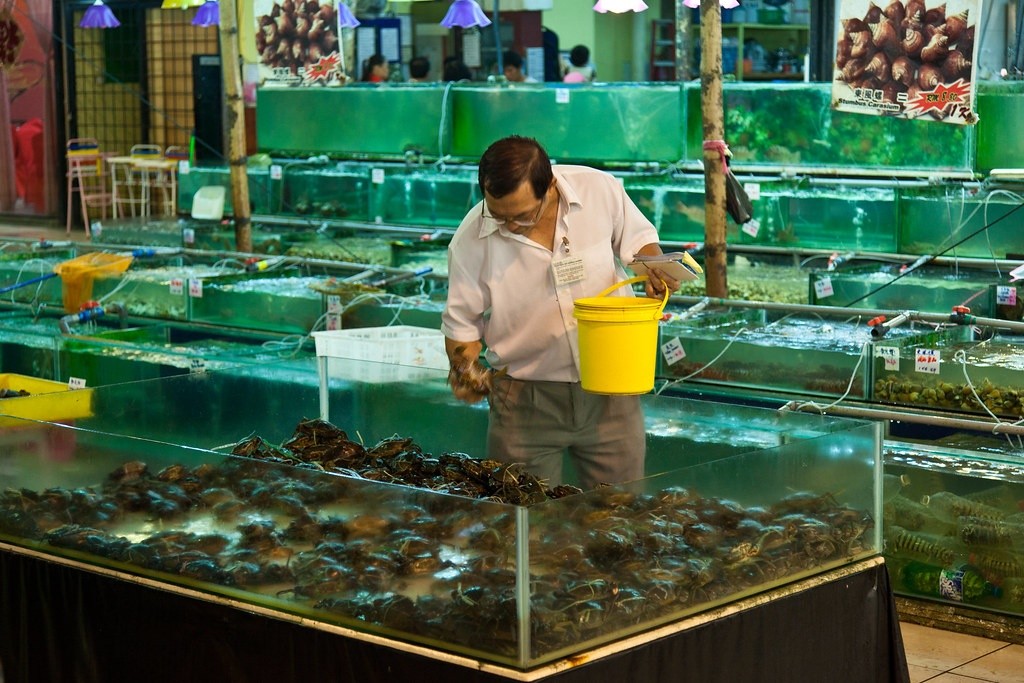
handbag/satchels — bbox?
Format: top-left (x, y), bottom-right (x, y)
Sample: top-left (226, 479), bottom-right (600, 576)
top-left (717, 145), bottom-right (752, 226)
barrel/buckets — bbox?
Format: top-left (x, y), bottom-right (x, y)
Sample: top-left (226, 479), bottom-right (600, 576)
top-left (574, 275), bottom-right (669, 393)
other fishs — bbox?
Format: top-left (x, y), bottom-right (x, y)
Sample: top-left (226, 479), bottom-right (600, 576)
top-left (639, 197), bottom-right (707, 226)
top-left (730, 144), bottom-right (802, 163)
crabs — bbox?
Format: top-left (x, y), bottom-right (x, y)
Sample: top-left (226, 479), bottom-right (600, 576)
top-left (446, 345), bottom-right (511, 413)
top-left (1, 418), bottom-right (872, 658)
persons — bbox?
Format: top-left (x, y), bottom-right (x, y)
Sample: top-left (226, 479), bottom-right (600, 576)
top-left (442, 136), bottom-right (679, 495)
top-left (359, 45), bottom-right (595, 82)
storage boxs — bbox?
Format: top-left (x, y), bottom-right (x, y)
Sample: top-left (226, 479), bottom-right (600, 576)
top-left (0, 70), bottom-right (1024, 682)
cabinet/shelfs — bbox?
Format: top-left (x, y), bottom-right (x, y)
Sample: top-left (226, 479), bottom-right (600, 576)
top-left (674, 22), bottom-right (812, 83)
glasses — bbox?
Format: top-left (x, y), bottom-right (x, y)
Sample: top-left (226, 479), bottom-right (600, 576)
top-left (481, 187), bottom-right (545, 226)
